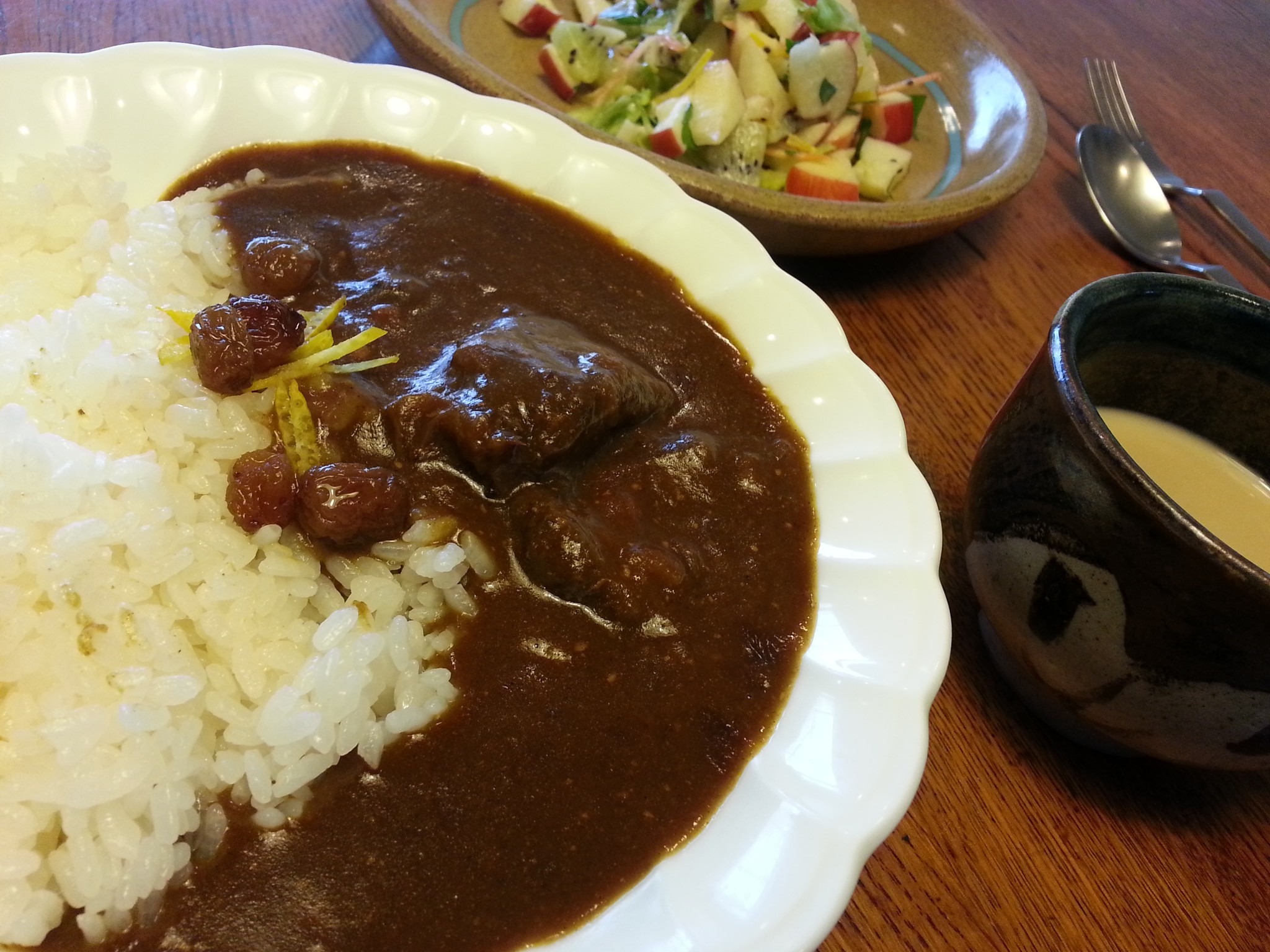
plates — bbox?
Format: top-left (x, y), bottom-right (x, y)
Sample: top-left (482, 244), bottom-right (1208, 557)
top-left (4, 39), bottom-right (952, 952)
top-left (361, 1), bottom-right (1050, 258)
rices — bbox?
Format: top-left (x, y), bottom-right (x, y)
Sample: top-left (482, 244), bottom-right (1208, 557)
top-left (4, 183), bottom-right (502, 947)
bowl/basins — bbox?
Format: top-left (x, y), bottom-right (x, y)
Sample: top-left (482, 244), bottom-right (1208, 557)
top-left (959, 270), bottom-right (1270, 784)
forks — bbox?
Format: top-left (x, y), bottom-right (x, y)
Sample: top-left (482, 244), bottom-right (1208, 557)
top-left (1081, 57), bottom-right (1269, 267)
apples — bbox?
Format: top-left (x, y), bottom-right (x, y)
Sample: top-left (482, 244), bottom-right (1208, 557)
top-left (496, 1), bottom-right (911, 203)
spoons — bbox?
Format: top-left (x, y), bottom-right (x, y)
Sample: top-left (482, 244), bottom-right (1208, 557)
top-left (1076, 124), bottom-right (1251, 292)
